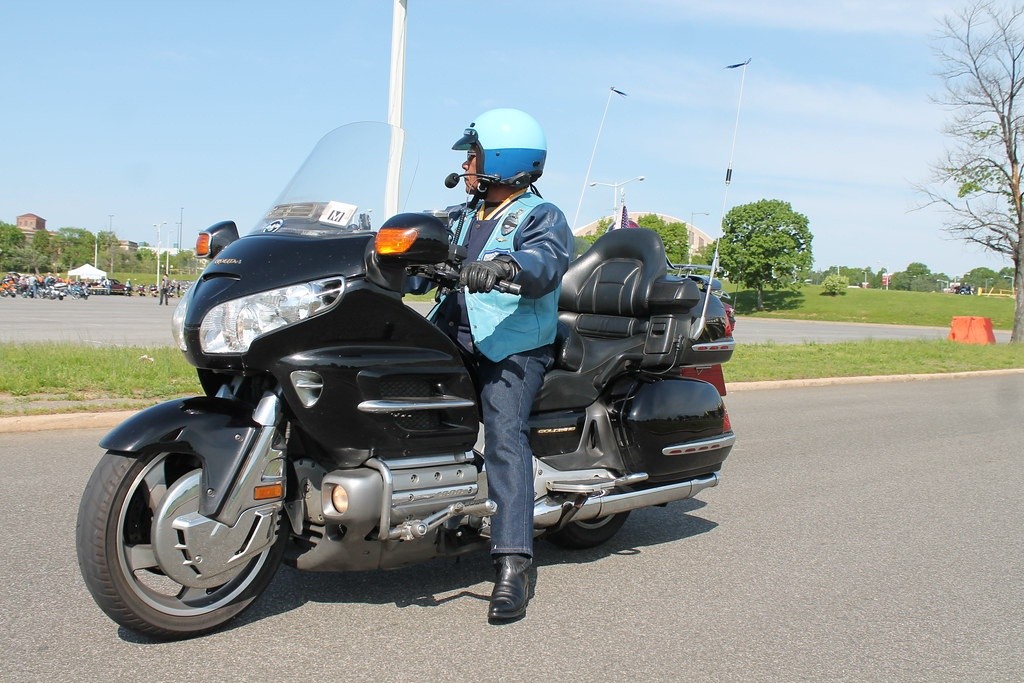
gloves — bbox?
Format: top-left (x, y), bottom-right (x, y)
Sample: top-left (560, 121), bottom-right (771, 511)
top-left (460, 254), bottom-right (520, 294)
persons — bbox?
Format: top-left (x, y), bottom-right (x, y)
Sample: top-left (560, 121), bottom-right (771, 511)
top-left (422, 107), bottom-right (574, 623)
top-left (105, 279), bottom-right (111, 295)
top-left (159, 274), bottom-right (170, 305)
top-left (125, 278), bottom-right (131, 296)
top-left (0, 272), bottom-right (70, 299)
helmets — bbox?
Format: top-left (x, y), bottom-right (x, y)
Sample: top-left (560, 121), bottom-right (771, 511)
top-left (451, 108), bottom-right (547, 191)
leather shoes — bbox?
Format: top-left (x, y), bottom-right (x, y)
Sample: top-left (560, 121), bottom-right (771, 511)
top-left (487, 553), bottom-right (537, 621)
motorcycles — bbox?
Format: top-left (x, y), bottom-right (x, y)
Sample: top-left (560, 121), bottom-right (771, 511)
top-left (75, 62), bottom-right (751, 642)
top-left (0, 270), bottom-right (93, 301)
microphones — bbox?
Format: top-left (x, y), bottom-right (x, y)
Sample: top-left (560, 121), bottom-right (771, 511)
top-left (445, 172), bottom-right (499, 188)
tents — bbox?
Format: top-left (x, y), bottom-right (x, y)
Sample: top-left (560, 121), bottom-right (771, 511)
top-left (68, 263), bottom-right (106, 281)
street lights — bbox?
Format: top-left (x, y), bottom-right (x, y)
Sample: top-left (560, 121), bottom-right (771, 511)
top-left (690, 212), bottom-right (710, 250)
top-left (985, 277), bottom-right (993, 293)
top-left (917, 274), bottom-right (929, 282)
top-left (862, 271), bottom-right (867, 288)
top-left (937, 280), bottom-right (949, 294)
top-left (882, 270), bottom-right (888, 290)
top-left (153, 222), bottom-right (169, 294)
top-left (589, 176), bottom-right (646, 222)
top-left (1004, 275), bottom-right (1015, 294)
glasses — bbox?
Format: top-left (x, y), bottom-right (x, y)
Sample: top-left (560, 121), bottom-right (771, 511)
top-left (466, 153), bottom-right (476, 162)
